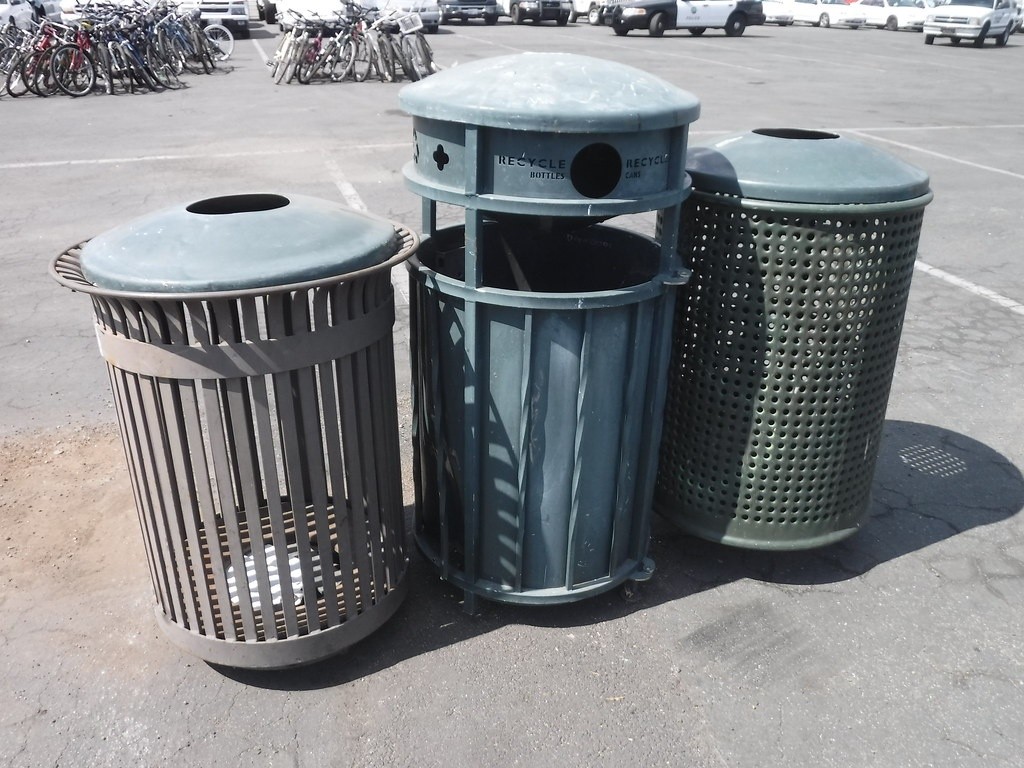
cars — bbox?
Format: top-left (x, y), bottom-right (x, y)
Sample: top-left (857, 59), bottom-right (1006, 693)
top-left (360, 0), bottom-right (442, 36)
top-left (191, 0), bottom-right (251, 40)
top-left (256, 0), bottom-right (354, 39)
top-left (922, 0), bottom-right (1017, 48)
top-left (441, 0), bottom-right (928, 47)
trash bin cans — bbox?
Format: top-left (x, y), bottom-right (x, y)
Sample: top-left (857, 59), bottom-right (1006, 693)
top-left (652, 127), bottom-right (934, 553)
top-left (47, 189), bottom-right (421, 673)
top-left (394, 51), bottom-right (702, 614)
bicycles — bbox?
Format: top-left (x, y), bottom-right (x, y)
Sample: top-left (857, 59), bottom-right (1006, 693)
top-left (0, 0), bottom-right (234, 99)
top-left (266, 0), bottom-right (440, 83)
